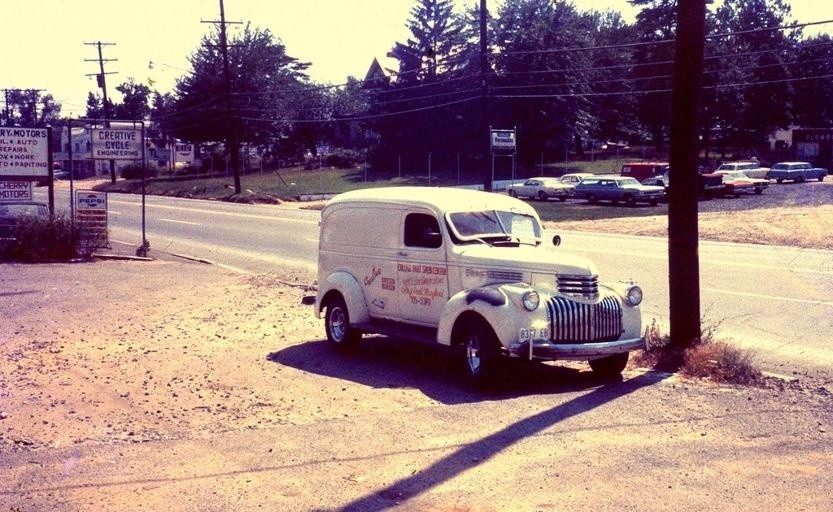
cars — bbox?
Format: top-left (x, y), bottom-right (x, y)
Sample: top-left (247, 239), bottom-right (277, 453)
top-left (766, 162), bottom-right (829, 183)
top-left (560, 160), bottom-right (771, 208)
top-left (505, 177), bottom-right (576, 202)
top-left (301, 184), bottom-right (653, 385)
